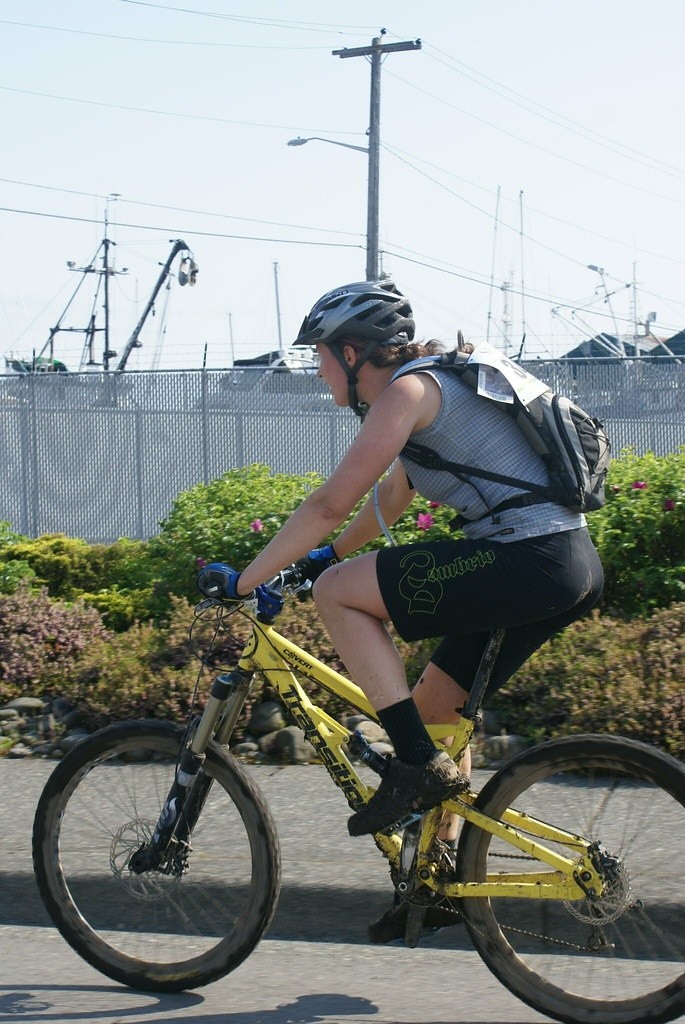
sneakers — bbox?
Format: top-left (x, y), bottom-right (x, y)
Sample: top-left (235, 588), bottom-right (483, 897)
top-left (347, 750), bottom-right (470, 836)
top-left (366, 876), bottom-right (464, 946)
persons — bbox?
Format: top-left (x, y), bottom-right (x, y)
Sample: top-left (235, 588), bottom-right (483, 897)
top-left (198, 281), bottom-right (608, 946)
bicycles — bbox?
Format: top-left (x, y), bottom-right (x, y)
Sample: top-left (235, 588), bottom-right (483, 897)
top-left (26, 555), bottom-right (685, 1022)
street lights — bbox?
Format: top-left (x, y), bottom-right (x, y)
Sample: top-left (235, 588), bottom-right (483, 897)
top-left (285, 134), bottom-right (380, 285)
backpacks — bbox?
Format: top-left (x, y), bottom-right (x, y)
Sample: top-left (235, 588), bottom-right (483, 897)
top-left (388, 349), bottom-right (612, 513)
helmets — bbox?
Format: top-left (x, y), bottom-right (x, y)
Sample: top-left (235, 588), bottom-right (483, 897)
top-left (293, 278), bottom-right (415, 345)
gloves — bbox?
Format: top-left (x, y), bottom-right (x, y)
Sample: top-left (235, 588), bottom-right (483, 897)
top-left (290, 542), bottom-right (341, 602)
top-left (195, 563), bottom-right (251, 608)
